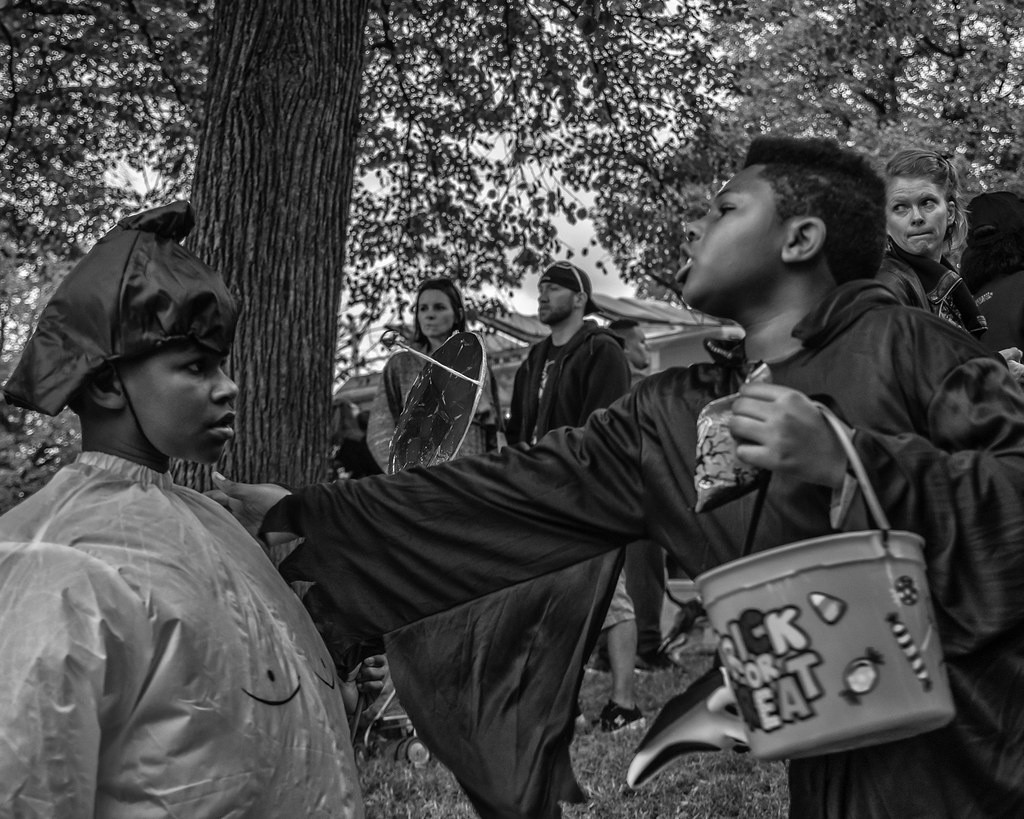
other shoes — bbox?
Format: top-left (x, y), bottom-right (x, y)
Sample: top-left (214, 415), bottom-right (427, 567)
top-left (588, 668), bottom-right (611, 680)
top-left (635, 664), bottom-right (681, 676)
top-left (590, 699), bottom-right (645, 735)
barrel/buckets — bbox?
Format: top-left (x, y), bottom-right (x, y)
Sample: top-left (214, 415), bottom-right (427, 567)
top-left (692, 402), bottom-right (956, 762)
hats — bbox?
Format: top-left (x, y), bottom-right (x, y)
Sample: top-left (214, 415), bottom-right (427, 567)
top-left (4, 199), bottom-right (236, 417)
top-left (538, 264), bottom-right (604, 316)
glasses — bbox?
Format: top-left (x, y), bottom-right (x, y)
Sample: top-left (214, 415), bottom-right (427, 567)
top-left (545, 260), bottom-right (583, 291)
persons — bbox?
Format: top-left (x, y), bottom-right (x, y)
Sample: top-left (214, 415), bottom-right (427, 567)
top-left (368, 279), bottom-right (506, 474)
top-left (885, 149), bottom-right (1024, 387)
top-left (508, 261), bottom-right (689, 736)
top-left (206, 136), bottom-right (1024, 819)
top-left (326, 397), bottom-right (384, 481)
top-left (0, 202), bottom-right (389, 819)
